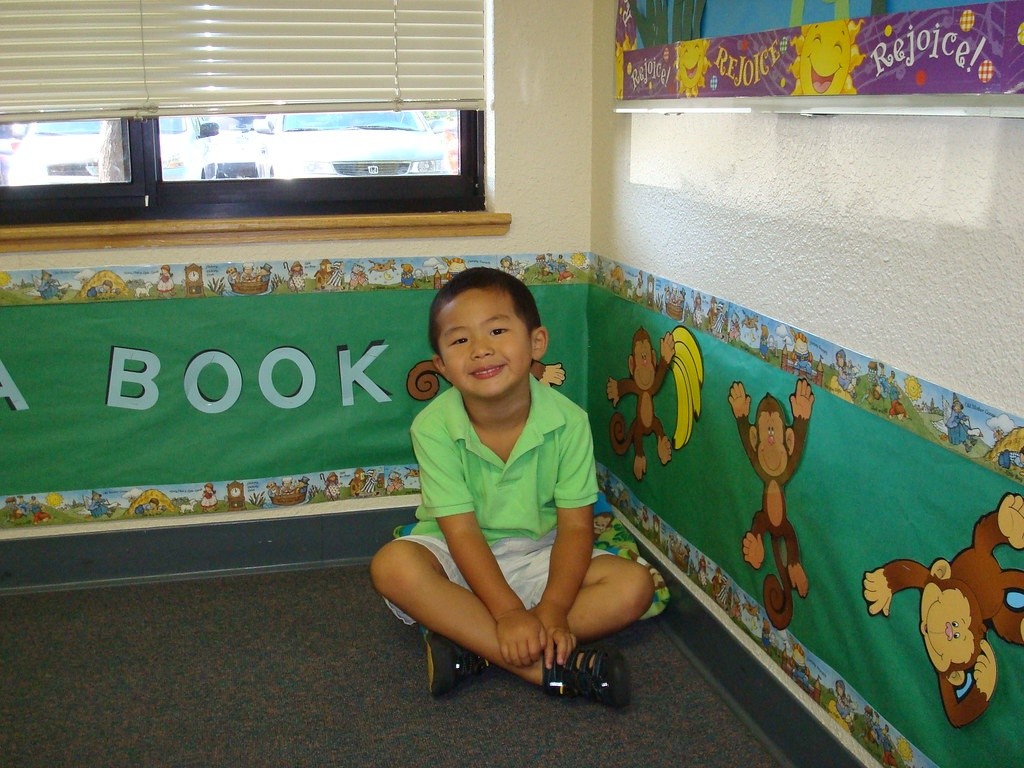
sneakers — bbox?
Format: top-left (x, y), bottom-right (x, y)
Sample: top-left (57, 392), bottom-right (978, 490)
top-left (423, 629), bottom-right (485, 696)
top-left (543, 643), bottom-right (630, 709)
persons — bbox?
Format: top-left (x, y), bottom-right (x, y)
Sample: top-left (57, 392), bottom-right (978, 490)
top-left (369, 266), bottom-right (654, 709)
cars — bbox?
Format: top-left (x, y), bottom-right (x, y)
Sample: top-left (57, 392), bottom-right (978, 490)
top-left (253, 109), bottom-right (453, 180)
top-left (198, 113), bottom-right (276, 181)
top-left (8, 115), bottom-right (220, 187)
